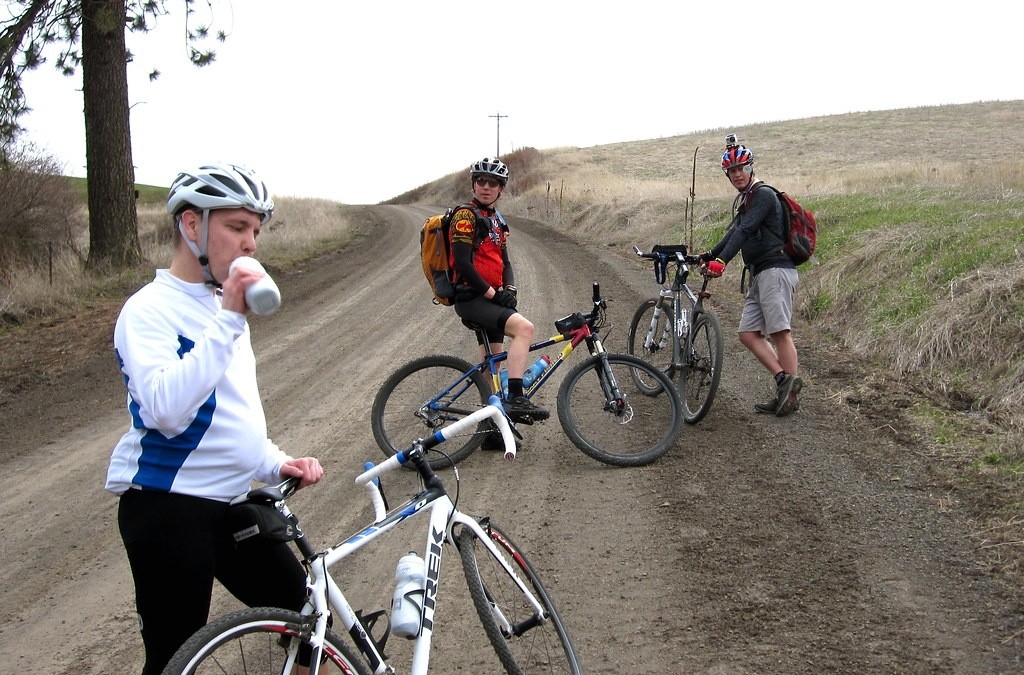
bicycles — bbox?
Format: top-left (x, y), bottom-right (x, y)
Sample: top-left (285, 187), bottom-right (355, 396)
top-left (626, 245), bottom-right (725, 425)
top-left (161, 394), bottom-right (582, 675)
top-left (371, 282), bottom-right (685, 468)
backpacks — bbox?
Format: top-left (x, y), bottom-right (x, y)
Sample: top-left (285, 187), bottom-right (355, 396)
top-left (750, 184), bottom-right (816, 265)
top-left (419, 204), bottom-right (505, 306)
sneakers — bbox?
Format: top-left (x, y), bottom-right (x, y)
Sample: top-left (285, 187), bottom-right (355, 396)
top-left (481, 431), bottom-right (523, 451)
top-left (504, 397), bottom-right (550, 421)
top-left (775, 375), bottom-right (802, 417)
top-left (755, 392), bottom-right (800, 412)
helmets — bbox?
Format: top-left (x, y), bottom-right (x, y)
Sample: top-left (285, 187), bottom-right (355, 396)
top-left (469, 159), bottom-right (509, 184)
top-left (166, 165), bottom-right (274, 225)
top-left (721, 144), bottom-right (753, 171)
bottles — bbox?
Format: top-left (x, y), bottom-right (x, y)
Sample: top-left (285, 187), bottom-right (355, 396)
top-left (520, 355), bottom-right (550, 389)
top-left (499, 367), bottom-right (508, 401)
top-left (228, 255), bottom-right (280, 322)
top-left (392, 551), bottom-right (427, 638)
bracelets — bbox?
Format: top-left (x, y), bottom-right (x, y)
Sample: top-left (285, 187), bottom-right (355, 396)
top-left (506, 285), bottom-right (516, 292)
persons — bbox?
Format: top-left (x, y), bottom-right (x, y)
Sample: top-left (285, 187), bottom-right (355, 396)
top-left (105, 163), bottom-right (332, 675)
top-left (450, 157), bottom-right (550, 452)
top-left (698, 133), bottom-right (802, 415)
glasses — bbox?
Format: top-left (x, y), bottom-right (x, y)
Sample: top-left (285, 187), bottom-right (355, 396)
top-left (475, 177), bottom-right (501, 188)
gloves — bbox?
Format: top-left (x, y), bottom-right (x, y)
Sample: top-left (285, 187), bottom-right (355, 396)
top-left (490, 289), bottom-right (518, 311)
